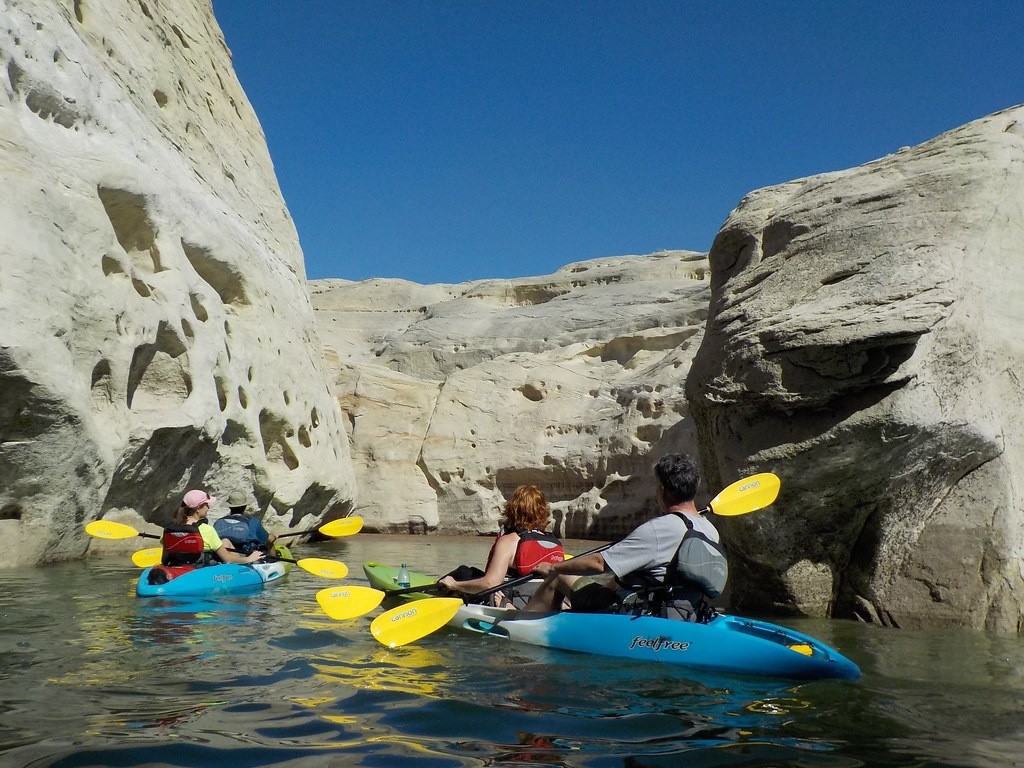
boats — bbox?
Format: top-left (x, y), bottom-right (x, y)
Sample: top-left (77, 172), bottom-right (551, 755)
top-left (362, 560), bottom-right (861, 682)
top-left (134, 544), bottom-right (294, 597)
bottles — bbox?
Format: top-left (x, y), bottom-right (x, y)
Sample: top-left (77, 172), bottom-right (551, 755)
top-left (398, 564), bottom-right (411, 589)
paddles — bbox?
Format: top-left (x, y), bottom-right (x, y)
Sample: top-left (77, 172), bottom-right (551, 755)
top-left (132, 515), bottom-right (364, 568)
top-left (83, 520), bottom-right (349, 580)
top-left (370, 472), bottom-right (780, 648)
top-left (316, 553), bottom-right (574, 621)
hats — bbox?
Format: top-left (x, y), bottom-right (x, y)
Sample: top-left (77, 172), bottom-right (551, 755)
top-left (183, 489), bottom-right (217, 508)
top-left (219, 492), bottom-right (254, 510)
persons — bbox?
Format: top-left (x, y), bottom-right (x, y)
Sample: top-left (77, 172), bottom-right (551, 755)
top-left (491, 453), bottom-right (720, 611)
top-left (214, 491), bottom-right (279, 557)
top-left (159, 490), bottom-right (267, 568)
top-left (438, 484), bottom-right (565, 596)
